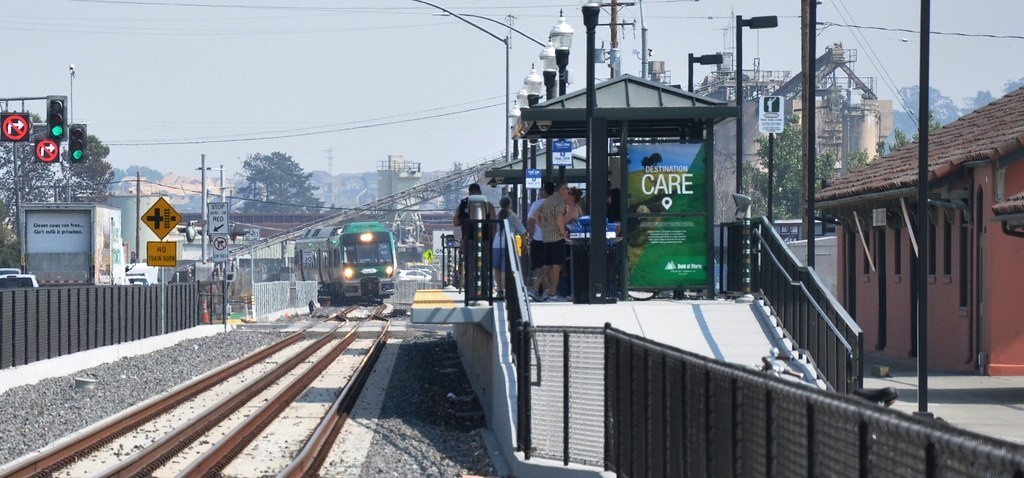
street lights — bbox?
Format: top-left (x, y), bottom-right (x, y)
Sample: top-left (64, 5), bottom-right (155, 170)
top-left (516, 85), bottom-right (529, 231)
top-left (507, 100), bottom-right (522, 216)
top-left (736, 14), bottom-right (779, 194)
top-left (549, 7), bottom-right (573, 190)
top-left (195, 167), bottom-right (227, 203)
top-left (524, 62), bottom-right (543, 204)
top-left (539, 38), bottom-right (556, 191)
top-left (687, 54), bottom-right (723, 92)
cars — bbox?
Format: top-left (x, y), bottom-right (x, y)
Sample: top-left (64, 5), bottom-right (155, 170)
top-left (125, 276), bottom-right (149, 285)
top-left (397, 269), bottom-right (432, 281)
top-left (0, 268), bottom-right (40, 289)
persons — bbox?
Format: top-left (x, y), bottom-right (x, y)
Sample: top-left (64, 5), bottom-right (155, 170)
top-left (453, 184), bottom-right (497, 296)
top-left (491, 197), bottom-right (527, 296)
top-left (525, 179), bottom-right (622, 302)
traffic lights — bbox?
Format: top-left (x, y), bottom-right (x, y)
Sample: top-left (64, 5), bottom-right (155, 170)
top-left (69, 124), bottom-right (87, 165)
top-left (46, 95), bottom-right (68, 142)
top-left (446, 235), bottom-right (455, 246)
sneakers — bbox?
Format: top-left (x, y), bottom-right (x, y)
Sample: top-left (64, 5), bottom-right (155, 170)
top-left (544, 294), bottom-right (566, 301)
top-left (528, 289), bottom-right (542, 301)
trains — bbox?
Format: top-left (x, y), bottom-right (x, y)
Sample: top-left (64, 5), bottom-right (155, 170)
top-left (295, 221), bottom-right (397, 303)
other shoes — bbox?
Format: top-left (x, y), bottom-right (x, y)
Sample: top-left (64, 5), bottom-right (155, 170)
top-left (496, 289), bottom-right (504, 297)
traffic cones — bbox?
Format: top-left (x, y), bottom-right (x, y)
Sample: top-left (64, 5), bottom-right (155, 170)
top-left (200, 299), bottom-right (209, 324)
